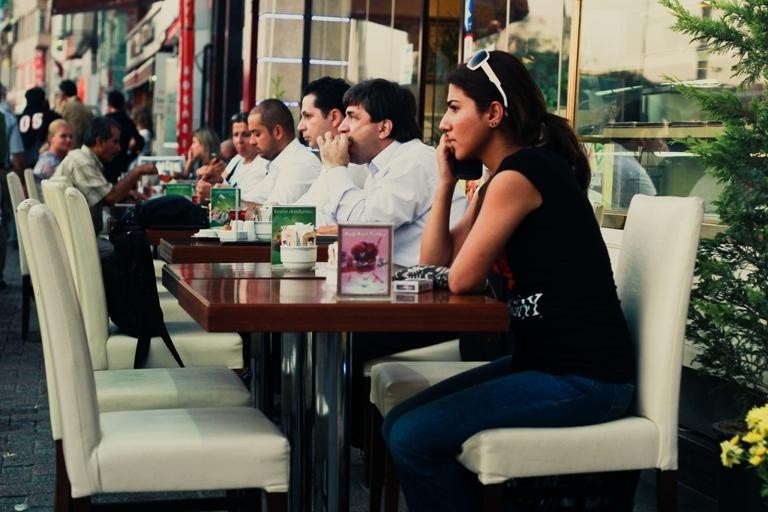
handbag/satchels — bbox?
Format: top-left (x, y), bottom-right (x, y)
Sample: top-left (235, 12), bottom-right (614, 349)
top-left (97, 227), bottom-right (163, 339)
top-left (392, 265), bottom-right (449, 287)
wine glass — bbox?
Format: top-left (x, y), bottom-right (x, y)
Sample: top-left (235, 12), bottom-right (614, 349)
top-left (353, 256), bottom-right (377, 287)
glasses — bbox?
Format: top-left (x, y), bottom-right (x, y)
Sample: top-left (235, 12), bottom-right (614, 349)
top-left (466, 49), bottom-right (508, 107)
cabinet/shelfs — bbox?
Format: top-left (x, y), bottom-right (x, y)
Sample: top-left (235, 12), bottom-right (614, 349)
top-left (597, 119), bottom-right (764, 247)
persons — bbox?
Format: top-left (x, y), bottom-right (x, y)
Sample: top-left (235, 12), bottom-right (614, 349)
top-left (0, 50), bottom-right (636, 512)
top-left (586, 107), bottom-right (657, 207)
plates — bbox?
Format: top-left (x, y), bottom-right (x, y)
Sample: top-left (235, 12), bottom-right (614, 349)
top-left (191, 234), bottom-right (219, 239)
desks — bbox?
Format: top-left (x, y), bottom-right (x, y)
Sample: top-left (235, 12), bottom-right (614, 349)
top-left (107, 176), bottom-right (511, 512)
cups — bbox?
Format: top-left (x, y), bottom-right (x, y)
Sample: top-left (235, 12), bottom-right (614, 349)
top-left (327, 241), bottom-right (339, 267)
top-left (142, 176), bottom-right (153, 198)
top-left (229, 220), bottom-right (272, 241)
top-left (198, 229), bottom-right (217, 238)
top-left (280, 246), bottom-right (317, 272)
